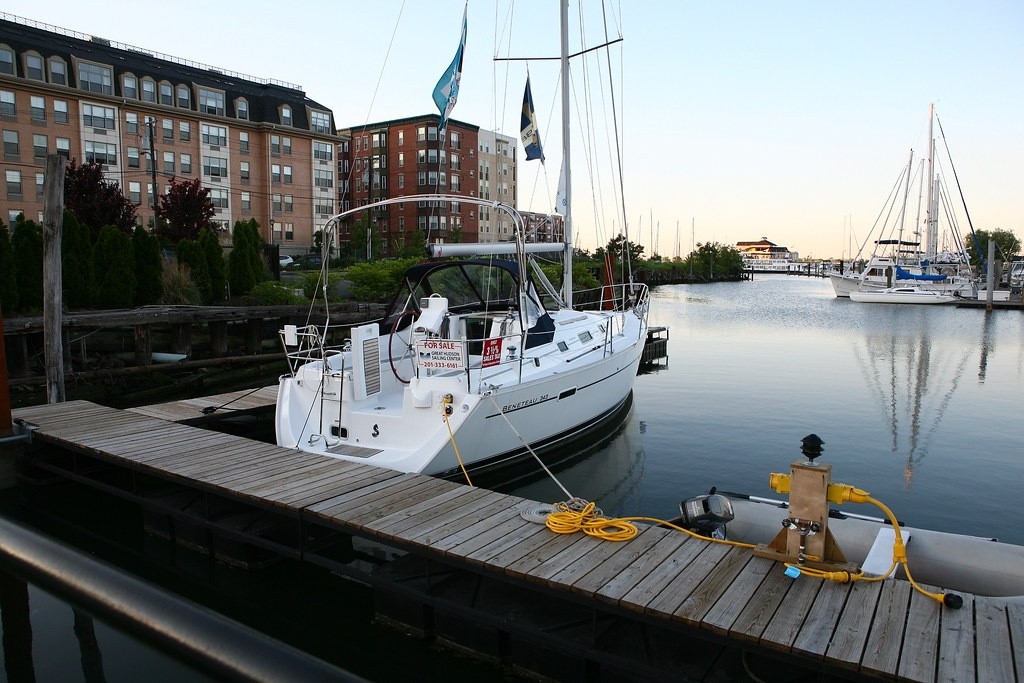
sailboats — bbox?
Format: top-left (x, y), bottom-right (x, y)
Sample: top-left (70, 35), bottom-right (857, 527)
top-left (274, 0), bottom-right (650, 477)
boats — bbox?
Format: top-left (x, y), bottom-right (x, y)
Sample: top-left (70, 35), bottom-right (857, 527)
top-left (822, 102), bottom-right (1024, 305)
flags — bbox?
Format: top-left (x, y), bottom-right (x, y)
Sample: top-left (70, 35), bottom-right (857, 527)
top-left (432, 5), bottom-right (467, 132)
top-left (520, 70), bottom-right (545, 164)
